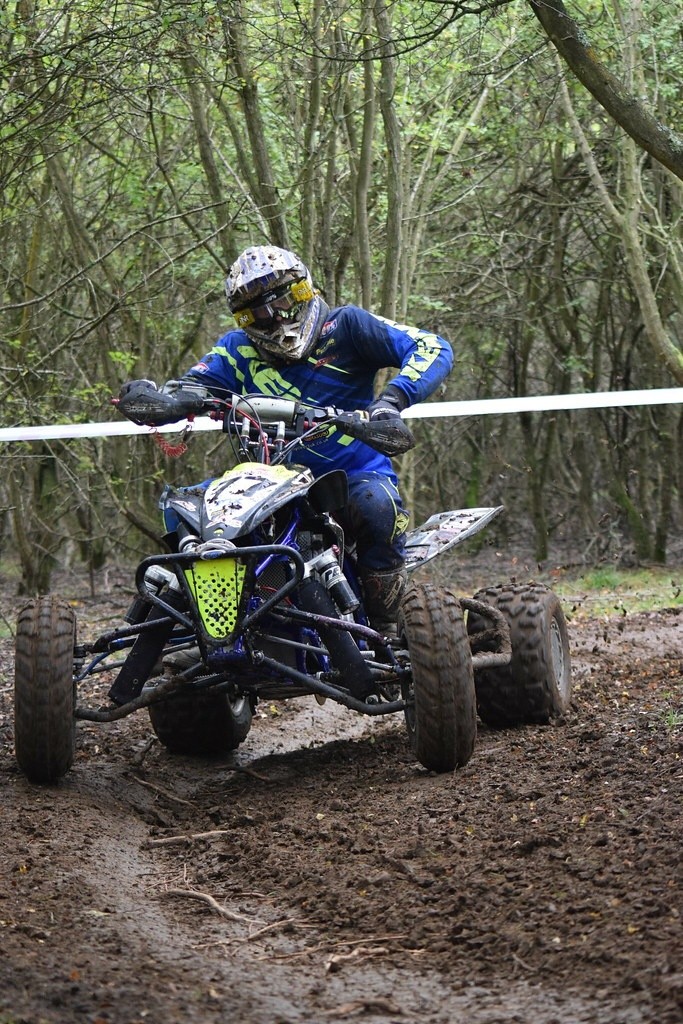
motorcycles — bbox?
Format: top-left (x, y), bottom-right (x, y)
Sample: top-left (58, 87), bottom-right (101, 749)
top-left (13, 377), bottom-right (571, 788)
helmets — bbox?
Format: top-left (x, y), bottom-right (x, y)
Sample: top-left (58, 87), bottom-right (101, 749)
top-left (223, 245), bottom-right (329, 367)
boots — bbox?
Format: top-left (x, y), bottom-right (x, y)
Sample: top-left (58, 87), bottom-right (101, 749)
top-left (361, 563), bottom-right (407, 650)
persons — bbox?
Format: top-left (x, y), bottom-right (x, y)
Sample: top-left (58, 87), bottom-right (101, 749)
top-left (113, 246), bottom-right (458, 666)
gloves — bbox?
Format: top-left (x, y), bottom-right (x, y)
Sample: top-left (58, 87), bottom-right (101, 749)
top-left (364, 385), bottom-right (410, 422)
top-left (119, 377), bottom-right (156, 398)
